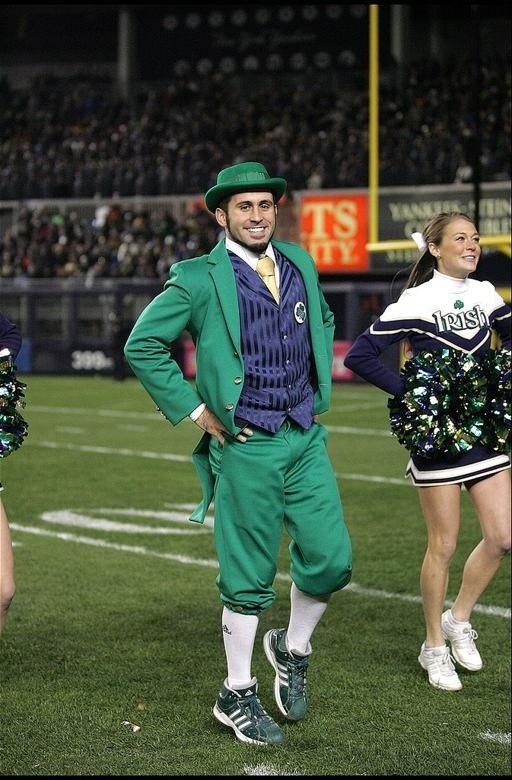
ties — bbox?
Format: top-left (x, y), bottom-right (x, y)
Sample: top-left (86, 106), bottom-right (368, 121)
top-left (256, 254), bottom-right (279, 306)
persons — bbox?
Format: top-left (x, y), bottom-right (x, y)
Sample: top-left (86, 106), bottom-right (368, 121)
top-left (0, 311), bottom-right (30, 628)
top-left (1, 0), bottom-right (512, 380)
top-left (343, 211), bottom-right (512, 692)
top-left (124, 161), bottom-right (355, 744)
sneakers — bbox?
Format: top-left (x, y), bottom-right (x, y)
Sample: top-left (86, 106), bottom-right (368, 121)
top-left (262, 629), bottom-right (311, 721)
top-left (418, 640), bottom-right (463, 691)
top-left (213, 679), bottom-right (284, 746)
top-left (441, 610), bottom-right (483, 672)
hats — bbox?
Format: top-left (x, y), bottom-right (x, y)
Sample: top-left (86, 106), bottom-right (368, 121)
top-left (204, 162), bottom-right (286, 215)
